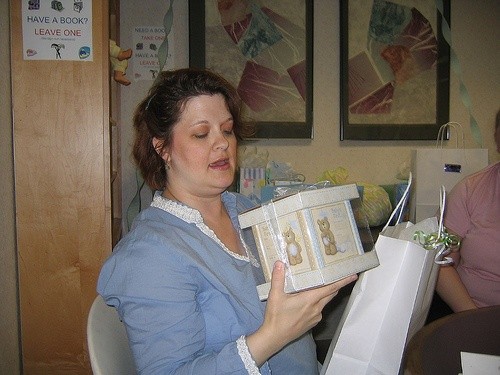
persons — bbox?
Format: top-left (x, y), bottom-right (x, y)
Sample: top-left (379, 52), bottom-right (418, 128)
top-left (109, 40), bottom-right (132, 86)
top-left (436, 111), bottom-right (500, 318)
top-left (97, 67), bottom-right (358, 375)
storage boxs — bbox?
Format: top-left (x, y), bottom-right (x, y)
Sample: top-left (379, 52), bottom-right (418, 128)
top-left (238, 183), bottom-right (379, 300)
top-left (240, 167), bottom-right (267, 199)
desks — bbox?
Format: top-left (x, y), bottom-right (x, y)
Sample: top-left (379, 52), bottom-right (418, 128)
top-left (402, 305), bottom-right (500, 375)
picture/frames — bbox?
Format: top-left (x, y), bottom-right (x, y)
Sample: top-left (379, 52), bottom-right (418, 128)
top-left (341, 0), bottom-right (451, 142)
top-left (188, 0), bottom-right (313, 140)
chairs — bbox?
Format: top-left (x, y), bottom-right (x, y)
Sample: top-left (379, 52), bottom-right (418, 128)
top-left (88, 294), bottom-right (139, 375)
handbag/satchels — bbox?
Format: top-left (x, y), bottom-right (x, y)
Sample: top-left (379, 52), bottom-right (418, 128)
top-left (321, 171), bottom-right (448, 375)
top-left (410, 121), bottom-right (489, 224)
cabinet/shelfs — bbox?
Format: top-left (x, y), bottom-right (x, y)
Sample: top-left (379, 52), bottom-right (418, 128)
top-left (9, 0), bottom-right (124, 375)
